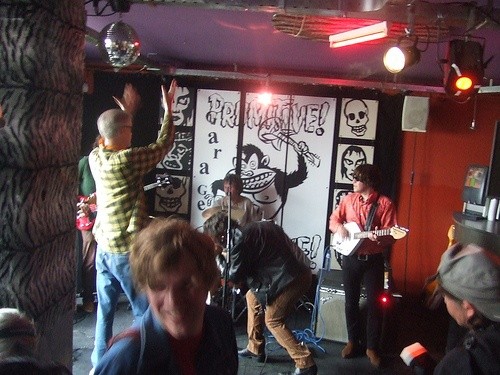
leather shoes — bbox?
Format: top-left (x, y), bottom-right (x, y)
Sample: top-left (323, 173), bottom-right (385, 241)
top-left (292, 364), bottom-right (317, 375)
top-left (238, 348), bottom-right (265, 362)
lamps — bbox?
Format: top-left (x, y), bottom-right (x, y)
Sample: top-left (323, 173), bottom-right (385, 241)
top-left (383, 1), bottom-right (432, 74)
top-left (328, 20), bottom-right (391, 49)
top-left (437, 30), bottom-right (494, 104)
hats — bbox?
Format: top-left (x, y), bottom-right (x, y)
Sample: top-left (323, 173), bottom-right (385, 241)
top-left (425, 242), bottom-right (500, 320)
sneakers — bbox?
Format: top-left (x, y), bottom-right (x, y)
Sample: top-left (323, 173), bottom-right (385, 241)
top-left (366, 346), bottom-right (382, 368)
top-left (341, 340), bottom-right (360, 359)
top-left (83, 299), bottom-right (95, 313)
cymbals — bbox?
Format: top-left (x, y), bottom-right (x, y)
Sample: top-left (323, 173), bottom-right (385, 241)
top-left (202, 206), bottom-right (245, 220)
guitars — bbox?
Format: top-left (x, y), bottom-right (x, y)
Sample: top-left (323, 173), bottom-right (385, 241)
top-left (76, 175), bottom-right (173, 231)
top-left (332, 222), bottom-right (409, 256)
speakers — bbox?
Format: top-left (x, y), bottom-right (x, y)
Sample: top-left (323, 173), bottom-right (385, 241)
top-left (401, 95), bottom-right (430, 132)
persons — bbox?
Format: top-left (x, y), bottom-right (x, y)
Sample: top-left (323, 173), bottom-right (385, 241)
top-left (203, 213), bottom-right (318, 375)
top-left (88, 79), bottom-right (177, 375)
top-left (202, 174), bottom-right (256, 232)
top-left (329, 163), bottom-right (398, 367)
top-left (0, 308), bottom-right (72, 375)
top-left (89, 215), bottom-right (239, 375)
top-left (433, 243), bottom-right (500, 375)
top-left (76, 135), bottom-right (102, 313)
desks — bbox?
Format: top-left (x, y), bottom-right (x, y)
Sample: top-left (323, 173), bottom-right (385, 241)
top-left (452, 211), bottom-right (500, 255)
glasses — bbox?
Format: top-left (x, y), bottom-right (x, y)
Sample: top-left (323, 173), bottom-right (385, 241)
top-left (353, 175), bottom-right (360, 181)
top-left (119, 125), bottom-right (136, 131)
